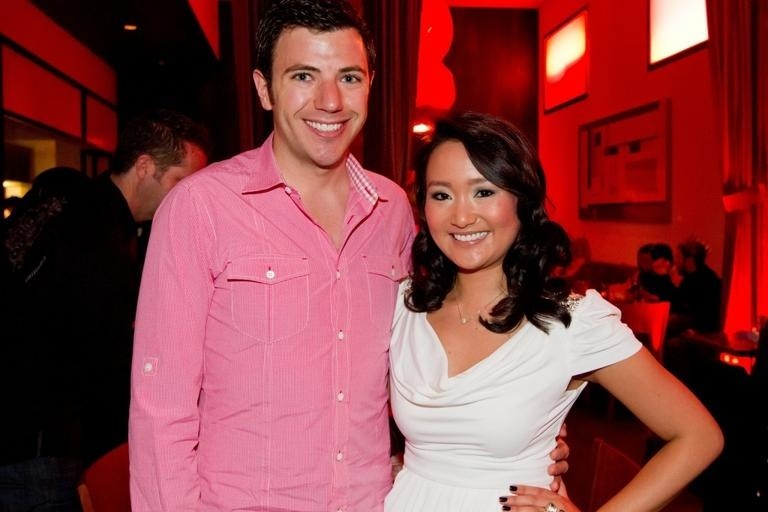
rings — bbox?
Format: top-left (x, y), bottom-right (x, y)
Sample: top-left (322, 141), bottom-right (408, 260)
top-left (544, 502), bottom-right (560, 512)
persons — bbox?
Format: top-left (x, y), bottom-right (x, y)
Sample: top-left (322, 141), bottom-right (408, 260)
top-left (626, 237), bottom-right (728, 351)
top-left (0, 105), bottom-right (210, 510)
top-left (382, 107), bottom-right (728, 512)
top-left (126, 1), bottom-right (571, 510)
top-left (446, 285), bottom-right (512, 328)
